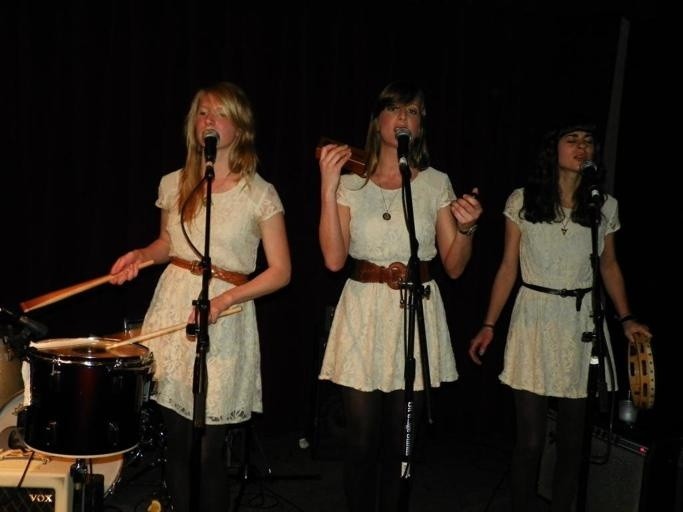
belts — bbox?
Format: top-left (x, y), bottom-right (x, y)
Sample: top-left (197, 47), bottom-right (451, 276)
top-left (169, 256), bottom-right (249, 286)
top-left (348, 258), bottom-right (435, 290)
top-left (522, 282), bottom-right (592, 312)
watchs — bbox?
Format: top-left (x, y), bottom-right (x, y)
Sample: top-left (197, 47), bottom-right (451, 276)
top-left (458, 225), bottom-right (479, 236)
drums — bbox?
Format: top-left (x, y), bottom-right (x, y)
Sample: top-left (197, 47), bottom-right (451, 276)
top-left (0, 387), bottom-right (124, 505)
top-left (17, 336), bottom-right (155, 458)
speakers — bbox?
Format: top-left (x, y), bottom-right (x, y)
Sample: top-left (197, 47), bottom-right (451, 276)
top-left (537, 407), bottom-right (678, 512)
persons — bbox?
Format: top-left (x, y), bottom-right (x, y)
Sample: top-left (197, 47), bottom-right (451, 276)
top-left (312, 78), bottom-right (485, 511)
top-left (109, 82), bottom-right (293, 512)
top-left (468, 111), bottom-right (655, 512)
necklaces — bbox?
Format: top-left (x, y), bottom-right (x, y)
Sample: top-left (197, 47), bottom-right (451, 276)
top-left (372, 164), bottom-right (406, 221)
top-left (549, 194), bottom-right (578, 236)
top-left (199, 168), bottom-right (232, 209)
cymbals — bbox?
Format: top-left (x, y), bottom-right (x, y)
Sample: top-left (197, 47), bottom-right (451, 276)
top-left (0, 308), bottom-right (49, 336)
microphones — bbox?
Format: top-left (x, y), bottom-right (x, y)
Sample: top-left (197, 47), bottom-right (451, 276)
top-left (581, 161), bottom-right (602, 209)
top-left (202, 128), bottom-right (220, 181)
top-left (394, 127), bottom-right (411, 180)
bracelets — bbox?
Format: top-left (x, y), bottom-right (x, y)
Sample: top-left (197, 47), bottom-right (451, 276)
top-left (482, 322), bottom-right (494, 329)
top-left (619, 315), bottom-right (634, 323)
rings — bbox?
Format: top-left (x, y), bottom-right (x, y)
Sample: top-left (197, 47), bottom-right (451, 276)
top-left (211, 320), bottom-right (218, 323)
